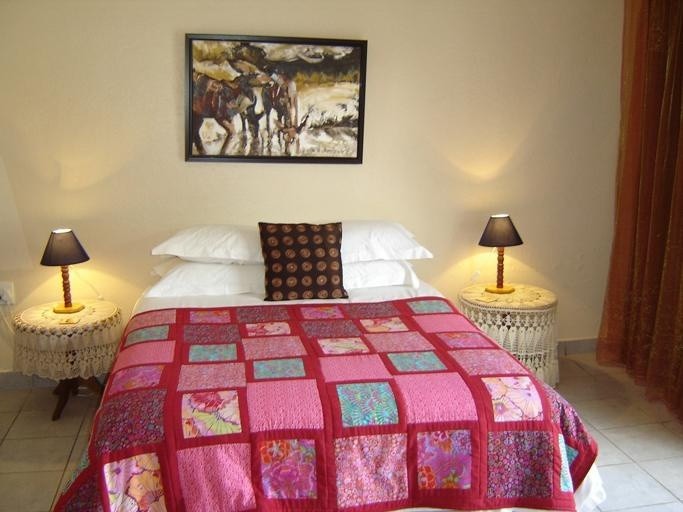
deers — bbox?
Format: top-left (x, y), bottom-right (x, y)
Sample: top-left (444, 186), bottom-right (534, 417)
top-left (191, 45), bottom-right (309, 157)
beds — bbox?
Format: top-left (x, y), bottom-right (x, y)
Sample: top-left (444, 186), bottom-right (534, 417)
top-left (96, 281), bottom-right (560, 512)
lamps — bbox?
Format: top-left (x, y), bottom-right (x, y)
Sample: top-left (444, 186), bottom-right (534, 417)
top-left (479, 213), bottom-right (524, 295)
top-left (39, 229), bottom-right (90, 314)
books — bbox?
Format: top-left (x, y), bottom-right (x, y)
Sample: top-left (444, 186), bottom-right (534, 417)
top-left (476, 296), bottom-right (500, 303)
top-left (59, 315), bottom-right (81, 325)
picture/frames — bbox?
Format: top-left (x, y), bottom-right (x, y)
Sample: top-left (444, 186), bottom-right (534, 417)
top-left (185, 34), bottom-right (368, 166)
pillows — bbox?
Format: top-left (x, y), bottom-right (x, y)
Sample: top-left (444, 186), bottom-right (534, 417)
top-left (146, 219), bottom-right (434, 299)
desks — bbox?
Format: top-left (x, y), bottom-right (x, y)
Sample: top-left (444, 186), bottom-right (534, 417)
top-left (460, 284), bottom-right (558, 370)
top-left (12, 301), bottom-right (122, 421)
